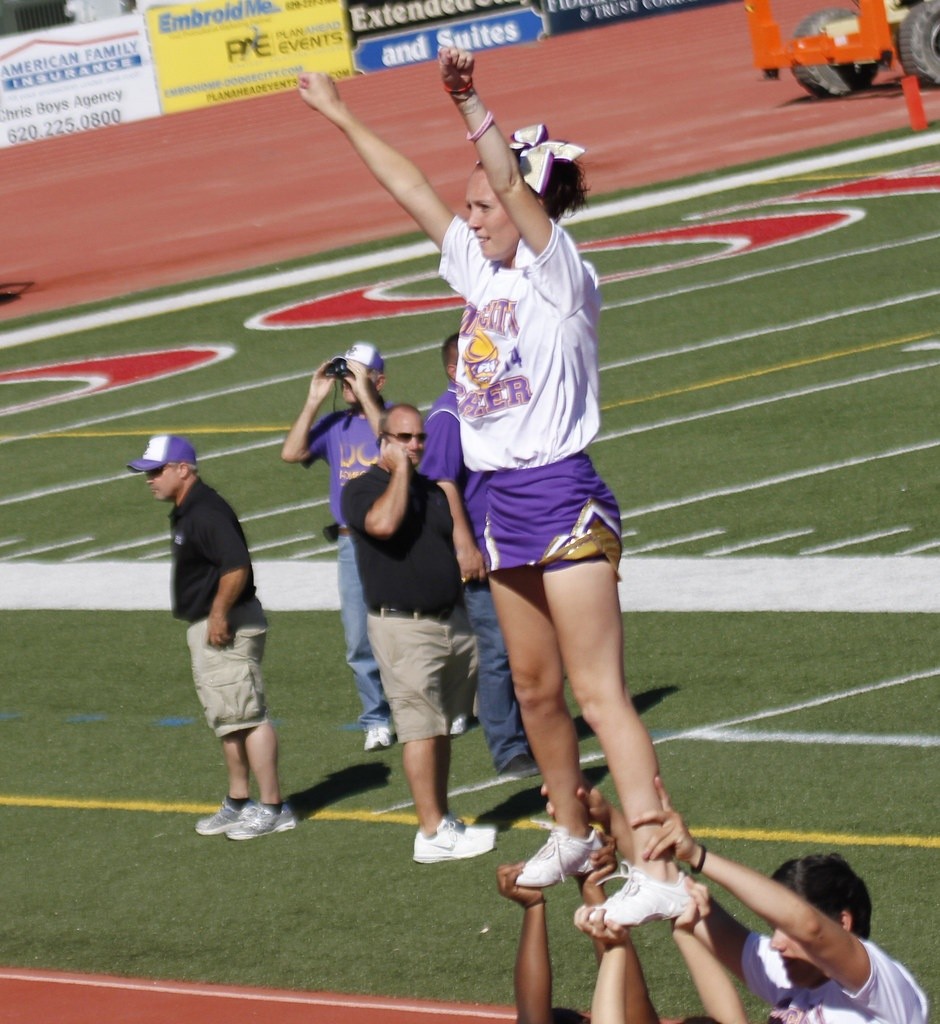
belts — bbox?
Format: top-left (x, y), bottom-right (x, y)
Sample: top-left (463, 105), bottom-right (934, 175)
top-left (368, 602), bottom-right (460, 621)
top-left (337, 527), bottom-right (351, 536)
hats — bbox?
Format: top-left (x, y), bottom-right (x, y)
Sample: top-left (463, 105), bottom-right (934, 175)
top-left (331, 342), bottom-right (384, 375)
top-left (127, 435), bottom-right (197, 473)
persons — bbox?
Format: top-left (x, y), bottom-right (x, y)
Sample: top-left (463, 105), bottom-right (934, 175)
top-left (294, 48), bottom-right (702, 928)
top-left (416, 333), bottom-right (542, 778)
top-left (340, 403), bottom-right (500, 864)
top-left (125, 435), bottom-right (296, 841)
top-left (281, 341), bottom-right (469, 752)
top-left (495, 774), bottom-right (928, 1024)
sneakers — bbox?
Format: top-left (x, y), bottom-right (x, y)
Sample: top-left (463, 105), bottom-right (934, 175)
top-left (194, 796), bottom-right (254, 835)
top-left (364, 726), bottom-right (391, 750)
top-left (515, 819), bottom-right (604, 887)
top-left (498, 755), bottom-right (540, 779)
top-left (225, 801), bottom-right (296, 840)
top-left (413, 813), bottom-right (496, 863)
top-left (450, 712), bottom-right (468, 735)
top-left (588, 861), bottom-right (692, 926)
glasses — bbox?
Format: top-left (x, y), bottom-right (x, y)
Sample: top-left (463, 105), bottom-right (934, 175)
top-left (150, 465), bottom-right (177, 477)
top-left (382, 430), bottom-right (427, 444)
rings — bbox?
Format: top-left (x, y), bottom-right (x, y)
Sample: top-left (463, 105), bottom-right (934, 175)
top-left (357, 369), bottom-right (359, 372)
top-left (461, 578), bottom-right (467, 582)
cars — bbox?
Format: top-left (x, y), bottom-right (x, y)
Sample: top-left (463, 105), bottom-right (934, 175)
top-left (739, 0), bottom-right (940, 102)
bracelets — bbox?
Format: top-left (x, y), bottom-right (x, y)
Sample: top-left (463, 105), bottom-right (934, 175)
top-left (444, 78), bottom-right (473, 94)
top-left (689, 845), bottom-right (706, 874)
top-left (466, 111), bottom-right (493, 141)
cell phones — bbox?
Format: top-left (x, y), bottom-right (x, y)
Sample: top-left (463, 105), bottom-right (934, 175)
top-left (375, 434), bottom-right (390, 450)
